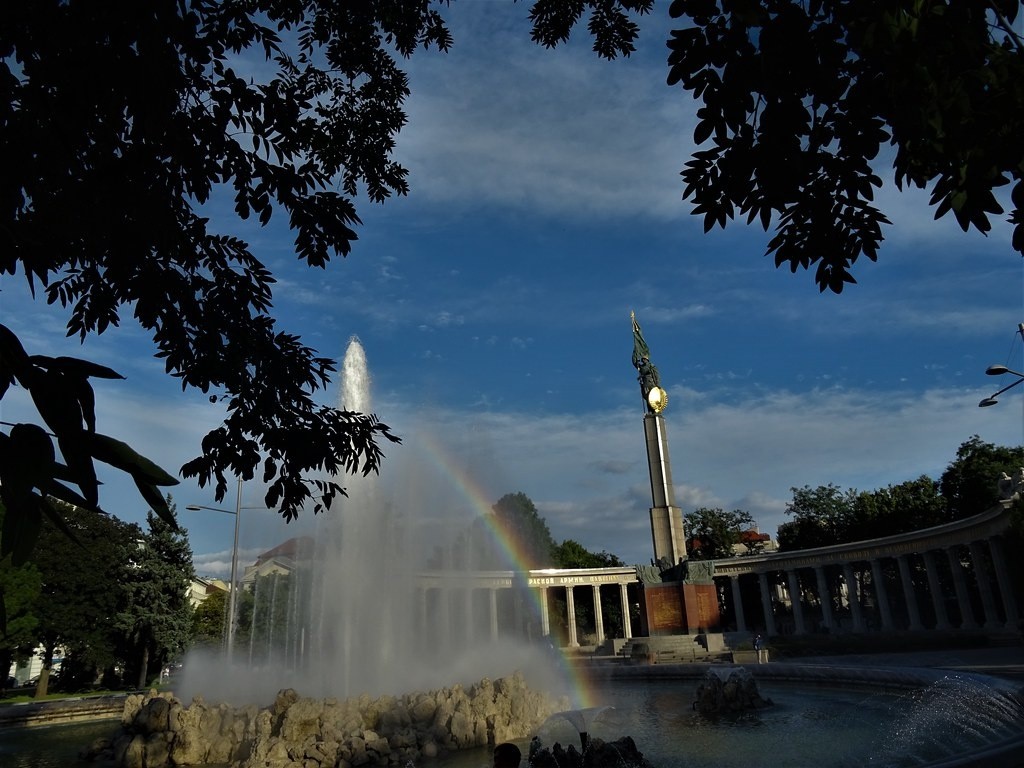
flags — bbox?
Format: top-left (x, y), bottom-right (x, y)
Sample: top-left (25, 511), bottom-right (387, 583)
top-left (632, 320), bottom-right (650, 361)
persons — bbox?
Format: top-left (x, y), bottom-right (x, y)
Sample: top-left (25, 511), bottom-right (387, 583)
top-left (753, 634), bottom-right (763, 664)
top-left (632, 349), bottom-right (661, 416)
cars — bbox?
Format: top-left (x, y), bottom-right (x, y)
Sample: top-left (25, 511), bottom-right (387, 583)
top-left (23, 674), bottom-right (57, 687)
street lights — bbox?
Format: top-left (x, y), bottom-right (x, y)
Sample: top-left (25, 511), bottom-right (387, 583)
top-left (185, 474), bottom-right (304, 668)
